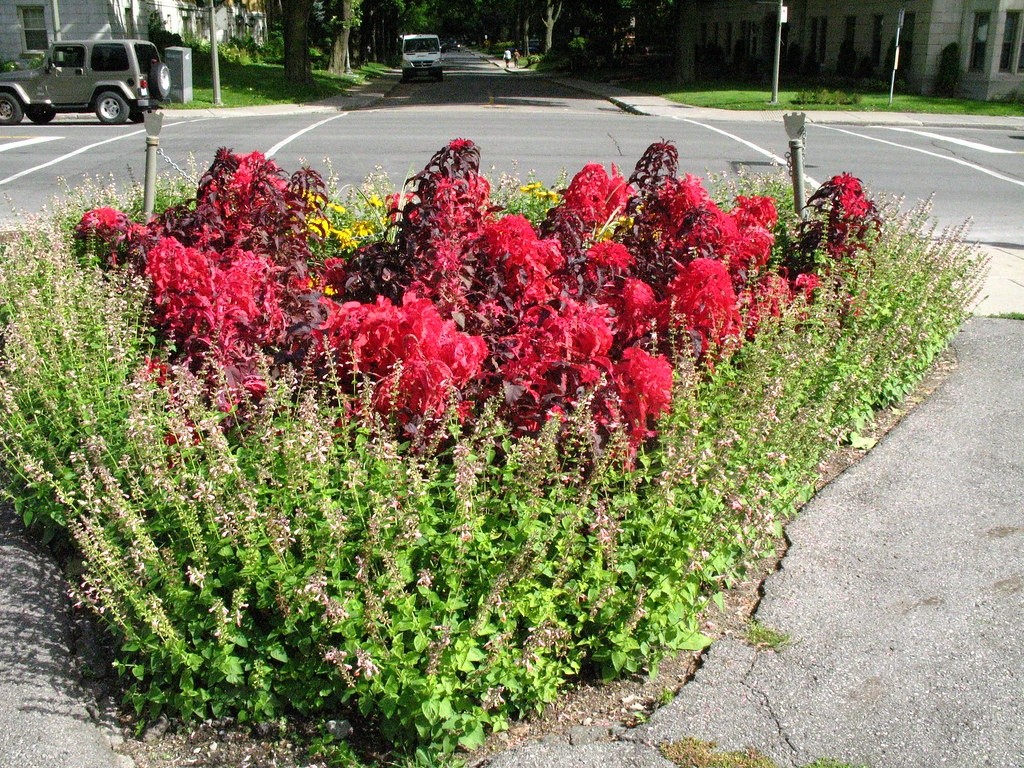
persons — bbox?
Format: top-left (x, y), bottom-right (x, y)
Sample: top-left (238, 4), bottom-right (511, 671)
top-left (503, 48), bottom-right (511, 69)
top-left (513, 48), bottom-right (521, 68)
top-left (439, 38), bottom-right (446, 61)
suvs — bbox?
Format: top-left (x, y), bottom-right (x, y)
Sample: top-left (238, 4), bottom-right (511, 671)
top-left (0, 39), bottom-right (171, 126)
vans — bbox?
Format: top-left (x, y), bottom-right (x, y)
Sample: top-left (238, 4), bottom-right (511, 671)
top-left (402, 34), bottom-right (444, 82)
